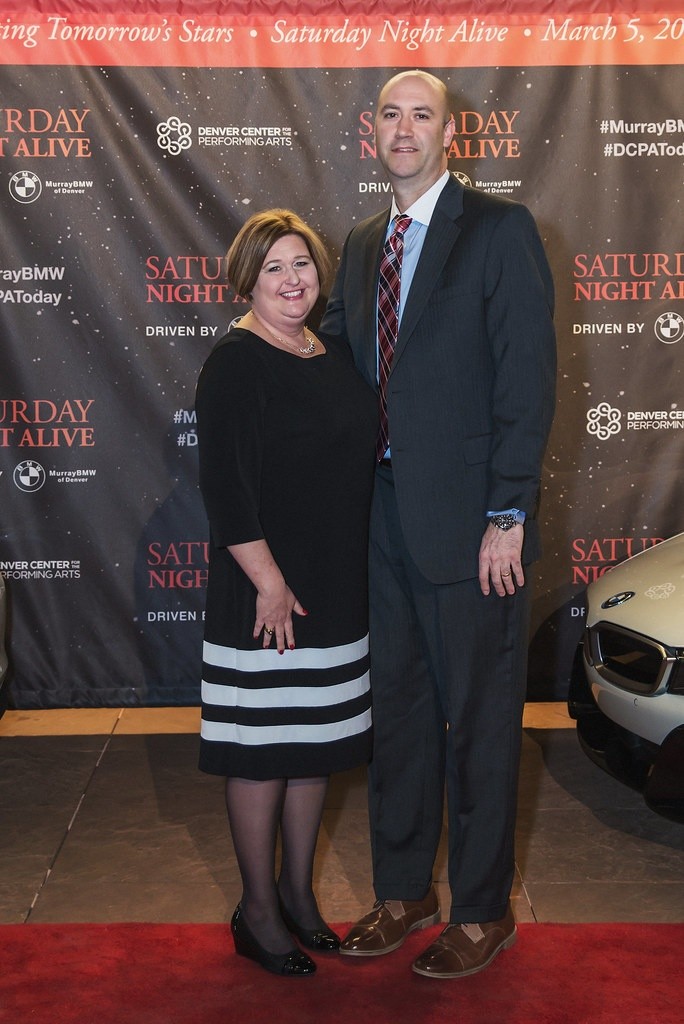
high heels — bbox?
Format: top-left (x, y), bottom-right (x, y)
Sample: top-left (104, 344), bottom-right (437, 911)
top-left (231, 903), bottom-right (317, 975)
top-left (277, 895), bottom-right (342, 951)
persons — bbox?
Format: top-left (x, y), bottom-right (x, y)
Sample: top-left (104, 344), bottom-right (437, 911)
top-left (194, 208), bottom-right (381, 977)
top-left (317, 70), bottom-right (557, 980)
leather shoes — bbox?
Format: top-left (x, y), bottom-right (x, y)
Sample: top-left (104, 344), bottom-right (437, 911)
top-left (411, 898), bottom-right (517, 978)
top-left (338, 883), bottom-right (441, 957)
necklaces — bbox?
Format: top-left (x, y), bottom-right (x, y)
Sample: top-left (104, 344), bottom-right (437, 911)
top-left (251, 310), bottom-right (315, 354)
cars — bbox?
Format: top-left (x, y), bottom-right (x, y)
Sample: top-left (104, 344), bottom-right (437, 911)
top-left (568, 533), bottom-right (684, 823)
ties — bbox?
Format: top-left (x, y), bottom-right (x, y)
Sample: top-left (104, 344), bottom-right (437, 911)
top-left (375, 213), bottom-right (413, 469)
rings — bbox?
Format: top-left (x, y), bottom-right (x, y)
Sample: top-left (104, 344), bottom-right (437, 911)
top-left (501, 573), bottom-right (510, 577)
top-left (264, 627), bottom-right (275, 635)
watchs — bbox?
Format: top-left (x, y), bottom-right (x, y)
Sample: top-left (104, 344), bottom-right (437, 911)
top-left (490, 514), bottom-right (521, 532)
